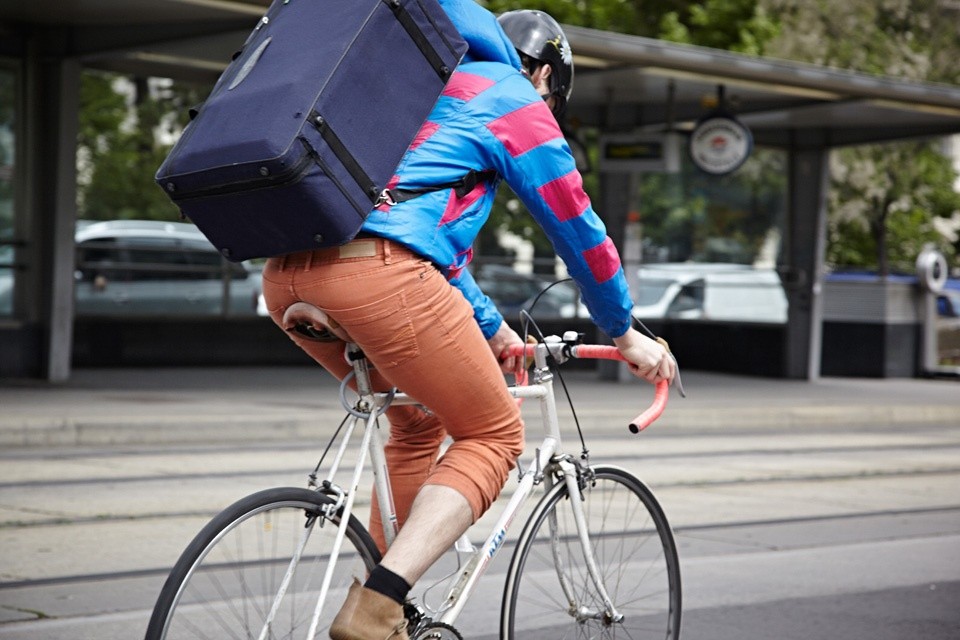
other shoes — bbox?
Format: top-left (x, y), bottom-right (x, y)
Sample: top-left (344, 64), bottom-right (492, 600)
top-left (328, 575), bottom-right (410, 640)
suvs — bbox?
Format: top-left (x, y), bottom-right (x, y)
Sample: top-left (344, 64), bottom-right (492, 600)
top-left (0, 216), bottom-right (259, 325)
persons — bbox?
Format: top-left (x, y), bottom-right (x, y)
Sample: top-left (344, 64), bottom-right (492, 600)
top-left (261, 0), bottom-right (675, 640)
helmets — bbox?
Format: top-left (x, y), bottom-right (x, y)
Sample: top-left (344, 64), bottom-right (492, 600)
top-left (497, 9), bottom-right (573, 120)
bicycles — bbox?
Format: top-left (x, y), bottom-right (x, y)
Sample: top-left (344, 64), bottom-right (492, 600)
top-left (144, 275), bottom-right (686, 640)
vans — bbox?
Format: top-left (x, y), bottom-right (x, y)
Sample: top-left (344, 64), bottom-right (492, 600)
top-left (633, 259), bottom-right (788, 324)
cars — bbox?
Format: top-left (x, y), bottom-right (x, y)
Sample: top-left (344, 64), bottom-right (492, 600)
top-left (465, 261), bottom-right (590, 322)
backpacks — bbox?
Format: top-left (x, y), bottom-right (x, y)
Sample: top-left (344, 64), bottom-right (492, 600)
top-left (154, 0), bottom-right (469, 263)
